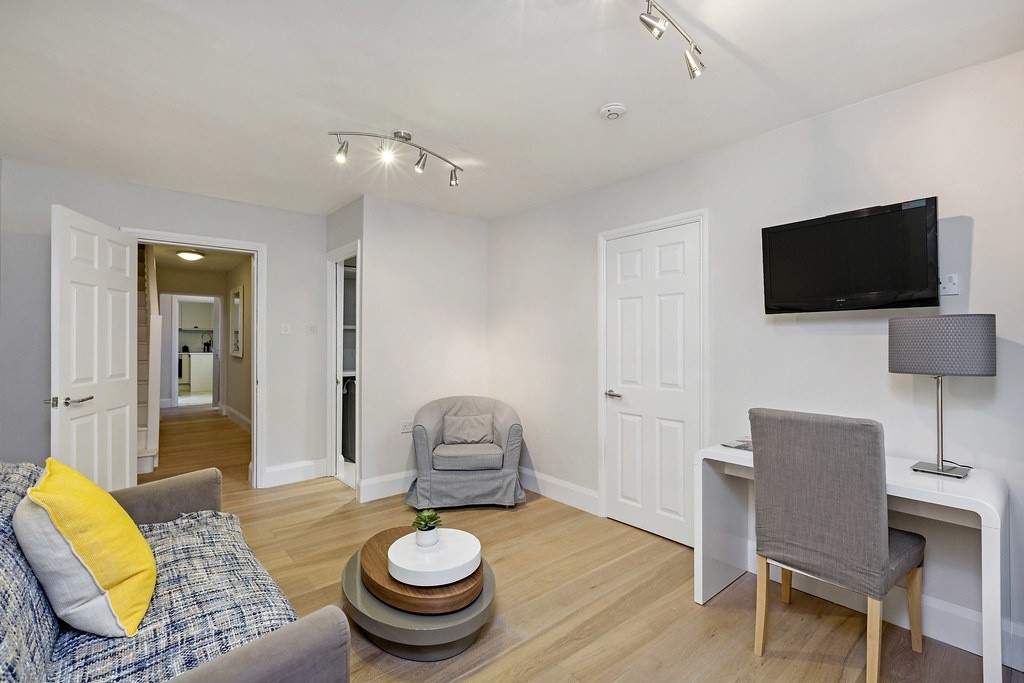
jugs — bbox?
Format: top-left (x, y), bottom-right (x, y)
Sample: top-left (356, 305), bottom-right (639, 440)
top-left (204, 343), bottom-right (209, 352)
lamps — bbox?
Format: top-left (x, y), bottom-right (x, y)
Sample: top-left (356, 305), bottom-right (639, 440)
top-left (639, 0), bottom-right (707, 80)
top-left (887, 314), bottom-right (998, 479)
top-left (328, 131), bottom-right (464, 187)
top-left (176, 251), bottom-right (205, 261)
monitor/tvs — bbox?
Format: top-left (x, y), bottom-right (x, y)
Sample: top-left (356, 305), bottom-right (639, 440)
top-left (762, 196), bottom-right (941, 315)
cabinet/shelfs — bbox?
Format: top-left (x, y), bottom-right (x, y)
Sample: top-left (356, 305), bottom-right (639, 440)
top-left (178, 300), bottom-right (214, 331)
top-left (181, 355), bottom-right (189, 378)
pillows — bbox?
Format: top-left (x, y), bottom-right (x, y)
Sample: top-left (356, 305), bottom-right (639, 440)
top-left (443, 415), bottom-right (494, 444)
top-left (11, 456), bottom-right (155, 639)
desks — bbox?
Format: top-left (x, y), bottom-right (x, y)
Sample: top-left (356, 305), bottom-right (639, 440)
top-left (341, 525), bottom-right (496, 662)
top-left (693, 432), bottom-right (1009, 683)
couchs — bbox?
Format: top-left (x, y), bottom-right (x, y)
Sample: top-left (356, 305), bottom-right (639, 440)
top-left (404, 395), bottom-right (527, 511)
top-left (0, 454), bottom-right (350, 683)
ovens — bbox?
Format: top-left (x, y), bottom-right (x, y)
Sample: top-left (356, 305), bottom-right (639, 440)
top-left (178, 354), bottom-right (190, 391)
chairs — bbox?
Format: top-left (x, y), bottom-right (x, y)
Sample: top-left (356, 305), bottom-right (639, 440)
top-left (748, 407), bottom-right (925, 683)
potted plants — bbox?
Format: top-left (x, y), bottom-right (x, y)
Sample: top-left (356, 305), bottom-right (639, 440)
top-left (411, 508), bottom-right (443, 547)
